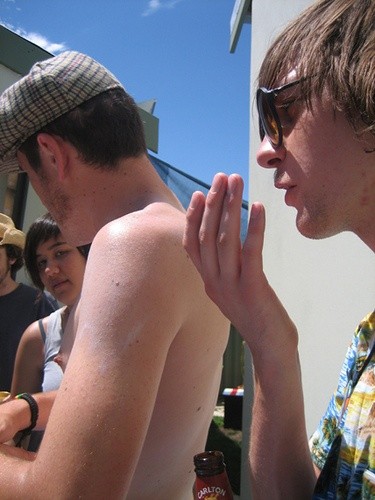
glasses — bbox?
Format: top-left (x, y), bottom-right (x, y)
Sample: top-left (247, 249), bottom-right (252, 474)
top-left (255, 63), bottom-right (334, 149)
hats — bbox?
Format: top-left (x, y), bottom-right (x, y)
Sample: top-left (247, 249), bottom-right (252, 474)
top-left (0, 213), bottom-right (26, 249)
top-left (0, 51), bottom-right (127, 177)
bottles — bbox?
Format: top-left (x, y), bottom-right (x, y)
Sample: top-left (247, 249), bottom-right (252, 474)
top-left (192, 451), bottom-right (233, 500)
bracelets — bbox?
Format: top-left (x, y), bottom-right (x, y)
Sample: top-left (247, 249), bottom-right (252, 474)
top-left (15, 392), bottom-right (40, 448)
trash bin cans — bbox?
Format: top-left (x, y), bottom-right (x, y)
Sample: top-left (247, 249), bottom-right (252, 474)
top-left (222, 388), bottom-right (243, 431)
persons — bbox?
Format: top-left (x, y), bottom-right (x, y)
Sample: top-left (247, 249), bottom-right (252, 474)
top-left (183, 0), bottom-right (375, 500)
top-left (9, 211), bottom-right (92, 456)
top-left (0, 212), bottom-right (54, 394)
top-left (0, 51), bottom-right (232, 500)
